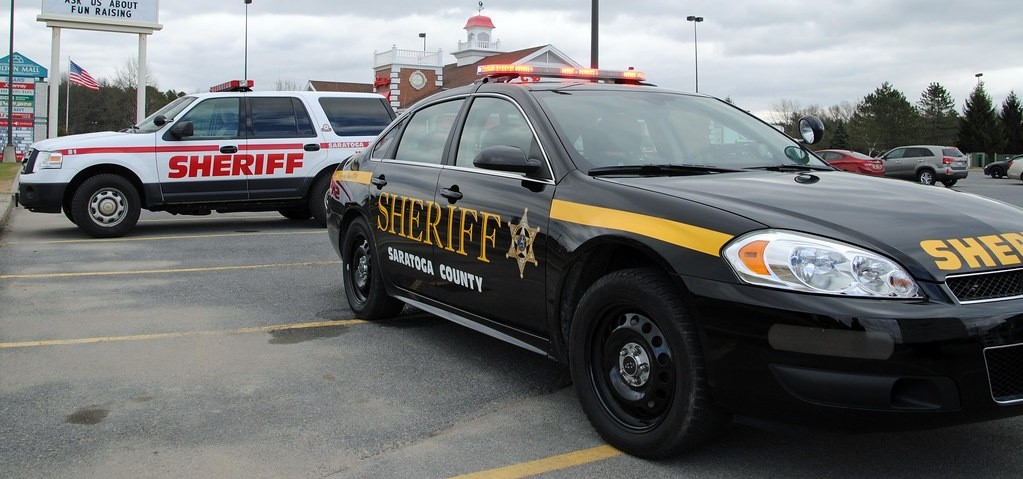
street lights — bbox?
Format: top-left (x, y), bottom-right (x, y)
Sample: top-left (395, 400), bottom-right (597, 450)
top-left (244, 0), bottom-right (255, 89)
top-left (975, 73), bottom-right (983, 89)
top-left (687, 15), bottom-right (704, 94)
top-left (419, 32), bottom-right (427, 56)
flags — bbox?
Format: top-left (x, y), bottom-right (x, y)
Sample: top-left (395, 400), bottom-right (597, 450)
top-left (69, 60), bottom-right (99, 91)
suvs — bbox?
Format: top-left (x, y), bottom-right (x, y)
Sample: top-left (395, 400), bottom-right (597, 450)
top-left (877, 145), bottom-right (969, 187)
top-left (14, 78), bottom-right (406, 229)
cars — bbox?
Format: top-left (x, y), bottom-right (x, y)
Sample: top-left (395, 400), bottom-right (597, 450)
top-left (323, 66), bottom-right (1023, 467)
top-left (984, 154), bottom-right (1021, 179)
top-left (1007, 157), bottom-right (1023, 180)
top-left (813, 149), bottom-right (884, 177)
